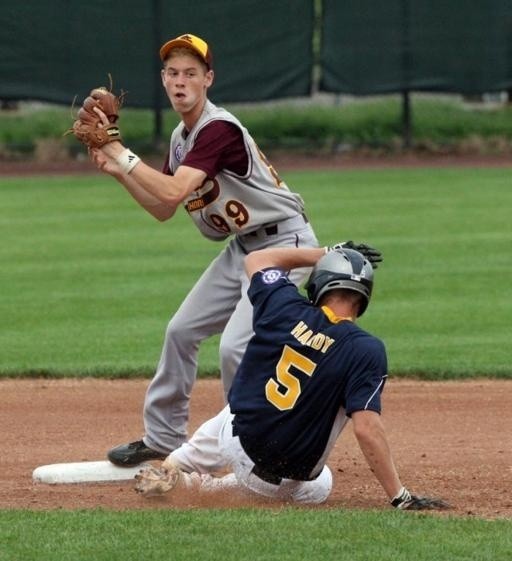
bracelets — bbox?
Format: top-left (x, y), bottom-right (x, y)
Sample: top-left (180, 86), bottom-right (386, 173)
top-left (117, 148), bottom-right (141, 175)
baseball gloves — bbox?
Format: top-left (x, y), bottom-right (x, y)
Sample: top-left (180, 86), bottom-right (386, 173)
top-left (64, 73), bottom-right (128, 148)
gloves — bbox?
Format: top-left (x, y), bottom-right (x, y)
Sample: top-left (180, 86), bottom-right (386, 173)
top-left (388, 485), bottom-right (457, 511)
top-left (324, 239), bottom-right (383, 269)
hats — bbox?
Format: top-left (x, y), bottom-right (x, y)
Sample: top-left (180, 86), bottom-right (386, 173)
top-left (159, 32), bottom-right (213, 71)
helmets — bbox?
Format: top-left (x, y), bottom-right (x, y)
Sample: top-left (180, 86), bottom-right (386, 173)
top-left (304, 247), bottom-right (375, 318)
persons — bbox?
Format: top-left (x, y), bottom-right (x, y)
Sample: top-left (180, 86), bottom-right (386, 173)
top-left (137, 240), bottom-right (449, 510)
top-left (70, 34), bottom-right (318, 468)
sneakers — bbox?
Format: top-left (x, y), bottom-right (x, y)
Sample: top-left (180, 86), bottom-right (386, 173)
top-left (131, 461), bottom-right (187, 502)
top-left (107, 436), bottom-right (170, 469)
